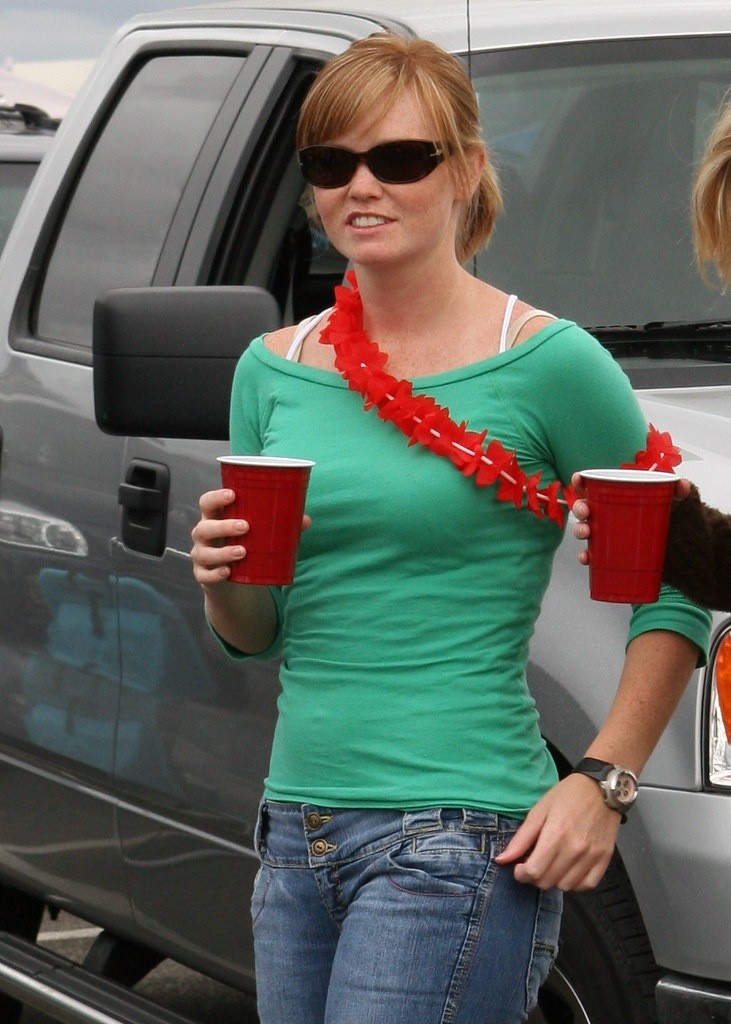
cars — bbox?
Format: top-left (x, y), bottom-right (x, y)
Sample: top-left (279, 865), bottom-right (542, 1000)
top-left (0, 104), bottom-right (55, 162)
top-left (0, 0), bottom-right (731, 1024)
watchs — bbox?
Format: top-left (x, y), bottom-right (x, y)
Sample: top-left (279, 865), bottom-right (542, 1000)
top-left (571, 757), bottom-right (638, 825)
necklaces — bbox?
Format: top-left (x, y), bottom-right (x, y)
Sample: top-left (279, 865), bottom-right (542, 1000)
top-left (318, 267), bottom-right (683, 528)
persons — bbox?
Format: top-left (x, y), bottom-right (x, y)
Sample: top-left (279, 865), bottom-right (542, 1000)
top-left (571, 102), bottom-right (731, 614)
top-left (190, 33), bottom-right (714, 1024)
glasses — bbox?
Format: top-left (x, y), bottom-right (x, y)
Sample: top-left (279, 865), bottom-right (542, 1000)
top-left (299, 140), bottom-right (452, 189)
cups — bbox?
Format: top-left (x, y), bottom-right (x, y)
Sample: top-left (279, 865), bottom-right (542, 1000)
top-left (217, 455), bottom-right (315, 586)
top-left (579, 469), bottom-right (681, 603)
